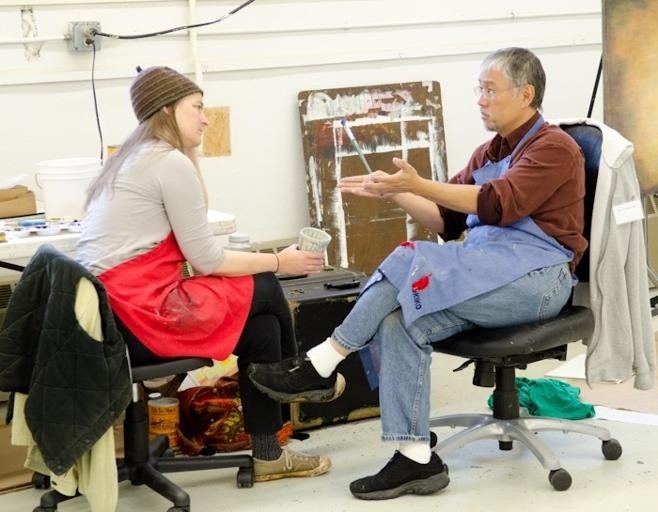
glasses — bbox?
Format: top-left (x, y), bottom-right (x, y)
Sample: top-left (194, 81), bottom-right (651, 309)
top-left (478, 81), bottom-right (527, 98)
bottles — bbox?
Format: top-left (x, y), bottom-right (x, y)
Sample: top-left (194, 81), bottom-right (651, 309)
top-left (227, 232), bottom-right (251, 252)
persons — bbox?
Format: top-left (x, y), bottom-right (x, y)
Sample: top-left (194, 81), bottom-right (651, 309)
top-left (71, 62), bottom-right (333, 481)
top-left (245, 47), bottom-right (589, 502)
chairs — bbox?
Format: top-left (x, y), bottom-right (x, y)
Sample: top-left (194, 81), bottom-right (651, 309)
top-left (432, 123), bottom-right (624, 491)
top-left (31, 242), bottom-right (256, 512)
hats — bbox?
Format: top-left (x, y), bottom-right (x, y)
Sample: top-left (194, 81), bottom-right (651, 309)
top-left (129, 64), bottom-right (204, 124)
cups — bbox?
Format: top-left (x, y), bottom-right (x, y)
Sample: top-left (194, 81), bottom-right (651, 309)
top-left (298, 227), bottom-right (332, 251)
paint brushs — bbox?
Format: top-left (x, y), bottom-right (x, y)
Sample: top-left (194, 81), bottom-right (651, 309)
top-left (341, 120), bottom-right (384, 197)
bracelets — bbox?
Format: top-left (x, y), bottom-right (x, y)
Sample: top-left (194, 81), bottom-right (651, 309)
top-left (273, 252), bottom-right (282, 274)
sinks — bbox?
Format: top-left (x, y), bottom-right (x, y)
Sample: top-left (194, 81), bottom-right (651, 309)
top-left (34, 158), bottom-right (101, 222)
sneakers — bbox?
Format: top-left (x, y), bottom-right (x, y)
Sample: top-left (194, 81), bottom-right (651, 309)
top-left (246, 352), bottom-right (347, 405)
top-left (437, 479), bottom-right (445, 482)
top-left (349, 451), bottom-right (449, 500)
top-left (250, 448), bottom-right (331, 484)
